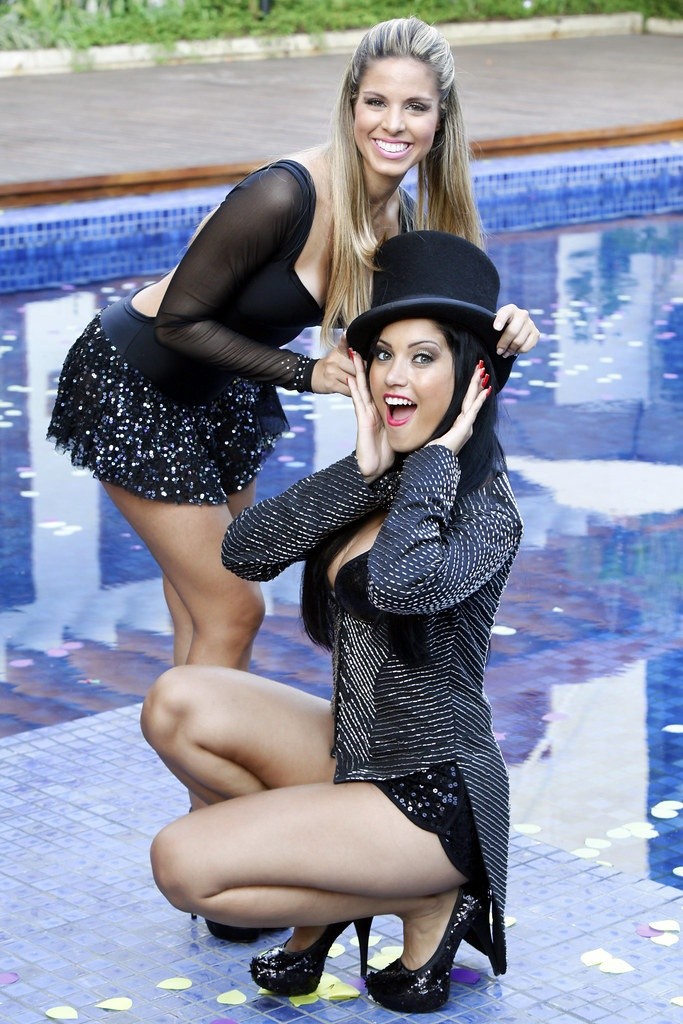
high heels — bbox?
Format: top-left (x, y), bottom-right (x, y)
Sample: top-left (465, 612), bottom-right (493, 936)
top-left (364, 884), bottom-right (500, 1013)
top-left (191, 912), bottom-right (288, 943)
top-left (249, 916), bottom-right (373, 994)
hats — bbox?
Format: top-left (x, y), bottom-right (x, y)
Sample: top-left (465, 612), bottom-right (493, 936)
top-left (347, 230), bottom-right (515, 394)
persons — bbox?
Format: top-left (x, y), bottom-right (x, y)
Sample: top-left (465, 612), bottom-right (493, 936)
top-left (47, 14), bottom-right (542, 943)
top-left (138, 229), bottom-right (523, 1014)
